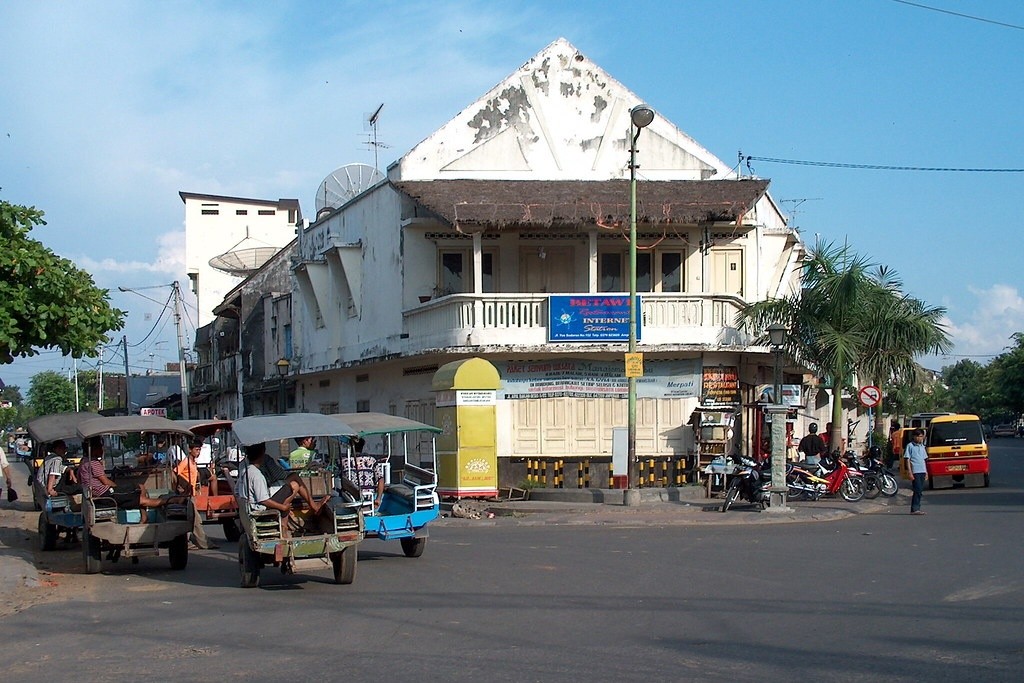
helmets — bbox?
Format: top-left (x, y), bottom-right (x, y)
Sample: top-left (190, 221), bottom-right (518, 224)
top-left (809, 423), bottom-right (817, 433)
top-left (870, 446), bottom-right (881, 457)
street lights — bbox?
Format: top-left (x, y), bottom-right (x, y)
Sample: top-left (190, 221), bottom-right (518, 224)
top-left (761, 322), bottom-right (792, 506)
top-left (81, 359), bottom-right (104, 409)
top-left (98, 341), bottom-right (133, 416)
top-left (622, 103), bottom-right (656, 506)
top-left (117, 285), bottom-right (191, 420)
top-left (275, 357), bottom-right (292, 459)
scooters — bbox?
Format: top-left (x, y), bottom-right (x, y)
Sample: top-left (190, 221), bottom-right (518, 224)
top-left (721, 441), bottom-right (897, 512)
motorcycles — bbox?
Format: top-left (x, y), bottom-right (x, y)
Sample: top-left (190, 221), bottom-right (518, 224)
top-left (5, 412), bottom-right (244, 551)
top-left (897, 414), bottom-right (990, 489)
top-left (320, 410), bottom-right (444, 557)
top-left (230, 412), bottom-right (364, 588)
top-left (73, 414), bottom-right (195, 576)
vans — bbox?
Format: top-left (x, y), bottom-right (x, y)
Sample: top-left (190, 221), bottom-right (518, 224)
top-left (907, 412), bottom-right (957, 445)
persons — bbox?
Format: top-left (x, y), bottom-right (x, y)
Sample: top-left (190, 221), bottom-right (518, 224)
top-left (903, 429), bottom-right (929, 515)
top-left (167, 434), bottom-right (243, 478)
top-left (238, 442), bottom-right (288, 484)
top-left (335, 437), bottom-right (385, 510)
top-left (798, 423), bottom-right (826, 464)
top-left (78, 437), bottom-right (170, 524)
top-left (818, 422), bottom-right (832, 458)
top-left (892, 422), bottom-right (901, 457)
top-left (0, 447), bottom-right (12, 497)
top-left (171, 437), bottom-right (221, 550)
top-left (37, 439), bottom-right (83, 497)
top-left (289, 435), bottom-right (319, 469)
top-left (233, 442), bottom-right (331, 539)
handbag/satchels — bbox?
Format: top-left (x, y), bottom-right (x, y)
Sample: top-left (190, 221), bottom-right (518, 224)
top-left (8, 484), bottom-right (18, 502)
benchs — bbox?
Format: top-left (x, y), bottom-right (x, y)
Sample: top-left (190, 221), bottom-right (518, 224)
top-left (156, 492), bottom-right (188, 523)
top-left (93, 495), bottom-right (118, 525)
top-left (386, 462), bottom-right (437, 510)
top-left (50, 491), bottom-right (71, 515)
top-left (322, 491), bottom-right (356, 537)
top-left (252, 507), bottom-right (283, 540)
top-left (196, 481), bottom-right (210, 496)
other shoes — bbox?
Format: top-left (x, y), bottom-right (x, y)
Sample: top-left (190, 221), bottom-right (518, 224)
top-left (188, 545), bottom-right (199, 550)
top-left (911, 509), bottom-right (925, 515)
top-left (203, 544), bottom-right (221, 549)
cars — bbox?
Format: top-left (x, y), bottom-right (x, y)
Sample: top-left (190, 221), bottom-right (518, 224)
top-left (982, 423), bottom-right (1016, 440)
